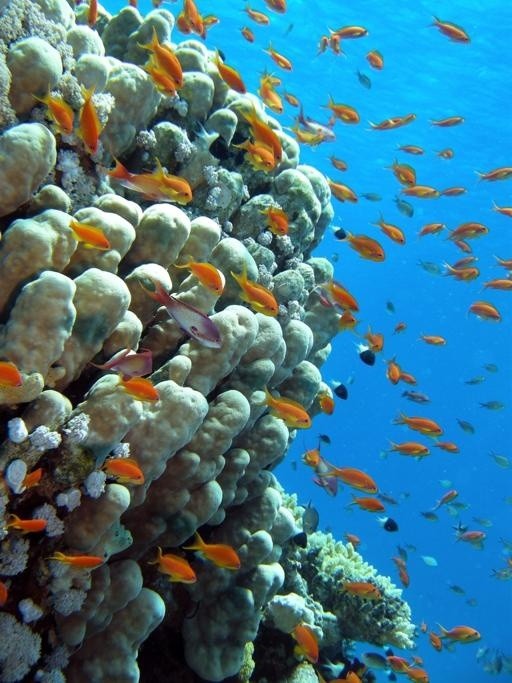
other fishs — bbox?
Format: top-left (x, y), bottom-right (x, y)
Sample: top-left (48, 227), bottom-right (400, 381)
top-left (0, 0), bottom-right (512, 683)
